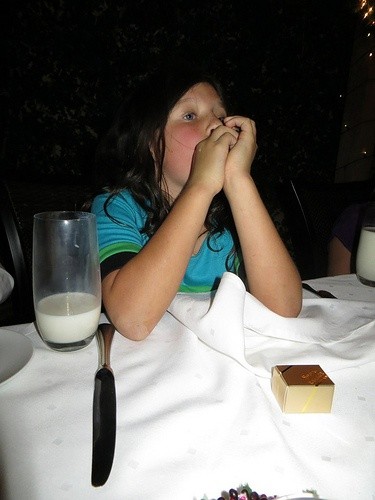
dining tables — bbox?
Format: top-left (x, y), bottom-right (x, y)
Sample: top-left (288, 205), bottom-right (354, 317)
top-left (0, 274), bottom-right (375, 500)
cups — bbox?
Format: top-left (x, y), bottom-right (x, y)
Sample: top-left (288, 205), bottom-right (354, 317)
top-left (32, 210), bottom-right (101, 353)
top-left (356, 207), bottom-right (375, 287)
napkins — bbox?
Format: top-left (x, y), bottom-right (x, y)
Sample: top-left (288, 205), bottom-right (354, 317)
top-left (0, 272), bottom-right (375, 500)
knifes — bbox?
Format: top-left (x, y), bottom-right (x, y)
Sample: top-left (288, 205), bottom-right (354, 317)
top-left (302, 283), bottom-right (337, 299)
top-left (91, 323), bottom-right (117, 487)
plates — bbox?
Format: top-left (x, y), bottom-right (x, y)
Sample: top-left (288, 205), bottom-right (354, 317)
top-left (0, 328), bottom-right (33, 386)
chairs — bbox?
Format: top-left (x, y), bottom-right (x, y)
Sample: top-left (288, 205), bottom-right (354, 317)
top-left (0, 175), bottom-right (375, 325)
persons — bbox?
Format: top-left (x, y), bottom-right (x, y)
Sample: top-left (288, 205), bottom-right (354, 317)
top-left (74, 60), bottom-right (302, 342)
